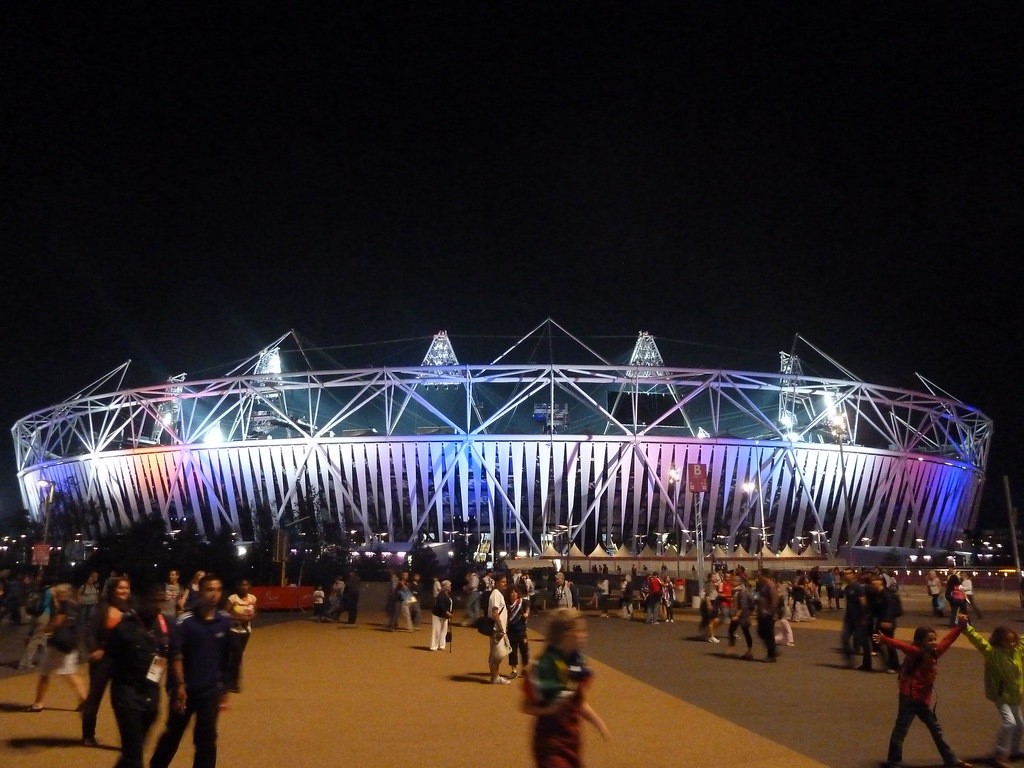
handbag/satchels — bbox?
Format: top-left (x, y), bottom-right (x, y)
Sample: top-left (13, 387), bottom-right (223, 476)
top-left (477, 616), bottom-right (495, 635)
top-left (653, 590), bottom-right (662, 599)
top-left (953, 586), bottom-right (965, 600)
top-left (399, 588), bottom-right (418, 605)
top-left (491, 632), bottom-right (513, 660)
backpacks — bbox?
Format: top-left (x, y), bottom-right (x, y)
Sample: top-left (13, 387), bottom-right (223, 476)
top-left (474, 575), bottom-right (486, 592)
top-left (520, 577), bottom-right (528, 597)
top-left (26, 585), bottom-right (51, 617)
top-left (747, 590), bottom-right (758, 611)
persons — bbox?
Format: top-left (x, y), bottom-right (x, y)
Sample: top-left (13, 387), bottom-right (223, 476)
top-left (756, 568), bottom-right (781, 664)
top-left (487, 575), bottom-right (512, 685)
top-left (224, 576), bottom-right (257, 694)
top-left (427, 558), bottom-right (793, 653)
top-left (79, 575), bottom-right (147, 748)
top-left (87, 582), bottom-right (185, 767)
top-left (27, 584), bottom-right (93, 711)
top-left (723, 574), bottom-right (755, 661)
top-left (959, 612), bottom-right (1024, 767)
top-left (150, 574), bottom-right (240, 768)
top-left (0, 557), bottom-right (209, 674)
top-left (505, 585), bottom-right (533, 681)
top-left (794, 557), bottom-right (983, 673)
top-left (521, 607), bottom-right (612, 767)
top-left (871, 615), bottom-right (978, 767)
top-left (311, 569), bottom-right (423, 633)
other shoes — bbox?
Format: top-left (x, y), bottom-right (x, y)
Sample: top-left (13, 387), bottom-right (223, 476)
top-left (886, 668), bottom-right (897, 674)
top-left (808, 617), bottom-right (815, 622)
top-left (75, 700), bottom-right (87, 712)
top-left (665, 619), bottom-right (670, 622)
top-left (793, 620), bottom-right (800, 623)
top-left (740, 653), bottom-right (753, 660)
top-left (19, 705), bottom-right (42, 713)
top-left (734, 633), bottom-right (742, 639)
top-left (491, 676), bottom-right (511, 685)
top-left (708, 636), bottom-right (720, 644)
top-left (653, 622), bottom-right (660, 624)
top-left (762, 656), bottom-right (776, 663)
top-left (508, 671), bottom-right (517, 679)
top-left (671, 619), bottom-right (674, 623)
top-left (520, 670), bottom-right (527, 678)
top-left (787, 642), bottom-right (795, 646)
